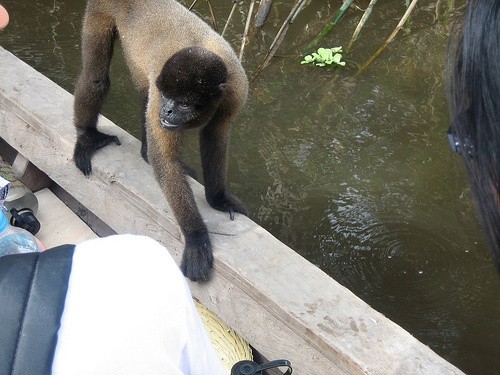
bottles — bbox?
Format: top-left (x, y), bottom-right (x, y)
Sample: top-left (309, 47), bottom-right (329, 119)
top-left (0, 157), bottom-right (40, 235)
top-left (0, 209), bottom-right (42, 260)
top-left (231, 359), bottom-right (292, 375)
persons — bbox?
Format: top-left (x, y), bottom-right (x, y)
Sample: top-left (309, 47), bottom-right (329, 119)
top-left (1, 1), bottom-right (225, 375)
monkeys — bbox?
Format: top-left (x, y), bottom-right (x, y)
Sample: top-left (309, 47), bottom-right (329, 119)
top-left (73, 0), bottom-right (250, 284)
